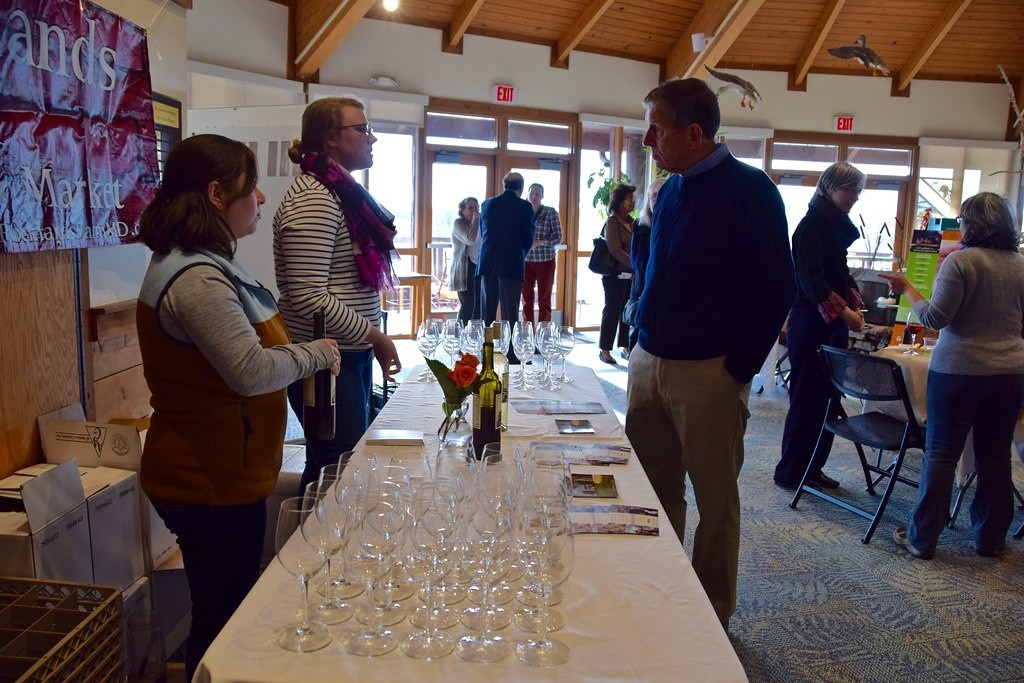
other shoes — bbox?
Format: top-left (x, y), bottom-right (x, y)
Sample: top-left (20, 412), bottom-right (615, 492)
top-left (774, 473), bottom-right (821, 495)
top-left (621, 350), bottom-right (629, 360)
top-left (599, 351), bottom-right (616, 364)
top-left (815, 469), bottom-right (841, 489)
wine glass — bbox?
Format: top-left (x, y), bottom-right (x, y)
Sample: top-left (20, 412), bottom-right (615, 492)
top-left (556, 326), bottom-right (575, 383)
top-left (535, 322), bottom-right (561, 391)
top-left (296, 480), bottom-right (354, 626)
top-left (401, 441), bottom-right (469, 661)
top-left (336, 452), bottom-right (434, 657)
top-left (315, 464), bottom-right (366, 599)
top-left (903, 312), bottom-right (923, 356)
top-left (514, 445), bottom-right (575, 667)
top-left (512, 321), bottom-right (536, 391)
top-left (417, 318), bottom-right (445, 383)
top-left (456, 442), bottom-right (520, 664)
top-left (442, 319), bottom-right (464, 371)
top-left (276, 497), bottom-right (332, 652)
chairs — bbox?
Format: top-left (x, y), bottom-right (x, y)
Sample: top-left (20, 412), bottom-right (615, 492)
top-left (789, 345), bottom-right (951, 544)
top-left (756, 321), bottom-right (792, 396)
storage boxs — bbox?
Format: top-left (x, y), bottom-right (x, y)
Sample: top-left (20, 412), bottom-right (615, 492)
top-left (0, 402), bottom-right (307, 683)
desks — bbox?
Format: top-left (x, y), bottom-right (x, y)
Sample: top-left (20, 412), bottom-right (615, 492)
top-left (863, 344), bottom-right (1024, 529)
top-left (189, 364), bottom-right (749, 683)
top-left (381, 272), bottom-right (432, 339)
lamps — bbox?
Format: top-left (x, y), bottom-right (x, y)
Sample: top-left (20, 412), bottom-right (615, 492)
top-left (691, 32), bottom-right (714, 53)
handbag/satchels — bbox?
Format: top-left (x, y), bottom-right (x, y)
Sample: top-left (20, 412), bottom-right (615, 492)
top-left (589, 216), bottom-right (618, 276)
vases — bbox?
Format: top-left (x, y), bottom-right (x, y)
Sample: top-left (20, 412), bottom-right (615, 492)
top-left (438, 398), bottom-right (472, 448)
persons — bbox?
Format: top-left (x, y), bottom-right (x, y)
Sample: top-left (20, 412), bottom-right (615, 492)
top-left (876, 192), bottom-right (1024, 560)
top-left (273, 97), bottom-right (401, 528)
top-left (623, 179), bottom-right (667, 353)
top-left (140, 135), bottom-right (342, 683)
top-left (625, 77), bottom-right (797, 636)
top-left (598, 184), bottom-right (635, 365)
top-left (773, 162), bottom-right (865, 495)
top-left (474, 173), bottom-right (532, 365)
top-left (335, 356), bottom-right (338, 363)
top-left (520, 183), bottom-right (562, 336)
top-left (449, 197), bottom-right (482, 358)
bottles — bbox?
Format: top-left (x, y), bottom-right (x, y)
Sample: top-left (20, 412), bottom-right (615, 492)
top-left (493, 323), bottom-right (509, 433)
top-left (473, 342), bottom-right (502, 461)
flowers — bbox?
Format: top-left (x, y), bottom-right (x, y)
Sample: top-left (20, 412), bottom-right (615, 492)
top-left (423, 350), bottom-right (480, 442)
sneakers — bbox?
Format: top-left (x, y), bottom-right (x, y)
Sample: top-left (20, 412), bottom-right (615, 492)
top-left (974, 544), bottom-right (1006, 558)
top-left (893, 526), bottom-right (935, 560)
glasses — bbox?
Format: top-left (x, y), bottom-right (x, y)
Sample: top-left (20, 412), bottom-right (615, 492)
top-left (956, 216), bottom-right (966, 224)
top-left (846, 186), bottom-right (861, 196)
top-left (341, 123), bottom-right (373, 134)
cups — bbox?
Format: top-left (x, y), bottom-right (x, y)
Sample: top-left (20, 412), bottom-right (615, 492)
top-left (460, 319), bottom-right (485, 355)
top-left (491, 320), bottom-right (511, 356)
top-left (923, 338), bottom-right (939, 351)
